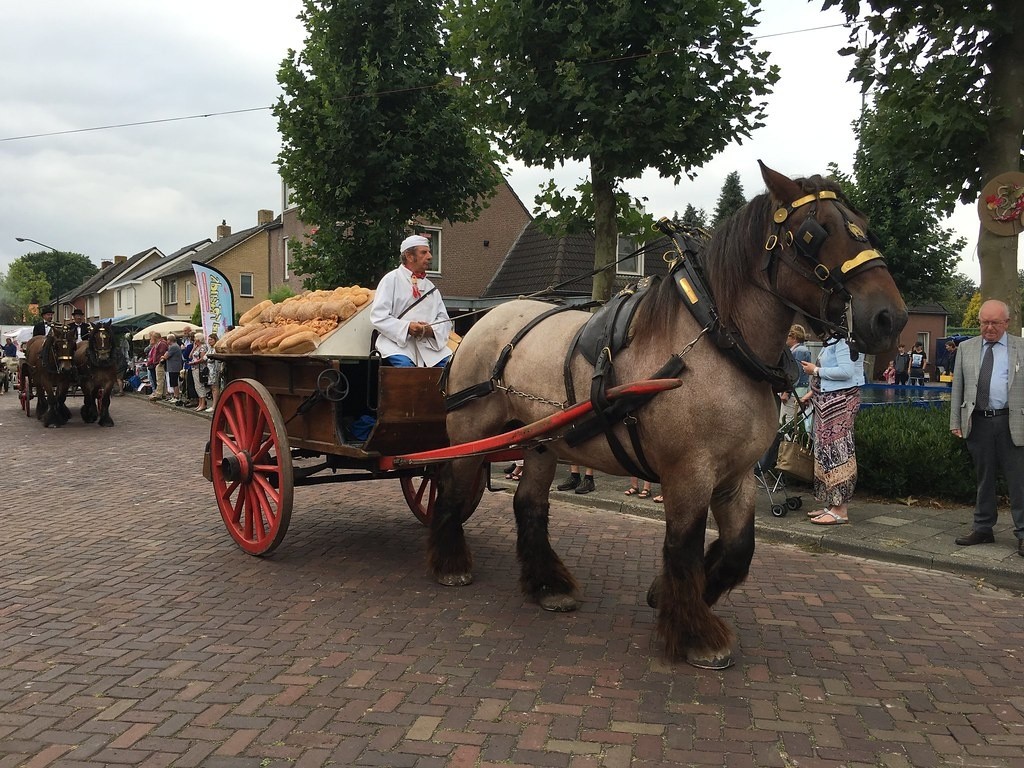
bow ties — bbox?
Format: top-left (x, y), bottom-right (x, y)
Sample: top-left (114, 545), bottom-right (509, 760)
top-left (76, 325), bottom-right (82, 327)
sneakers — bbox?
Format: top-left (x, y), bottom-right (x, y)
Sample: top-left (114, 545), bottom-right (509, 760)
top-left (955, 531), bottom-right (994, 545)
top-left (1018, 538), bottom-right (1024, 556)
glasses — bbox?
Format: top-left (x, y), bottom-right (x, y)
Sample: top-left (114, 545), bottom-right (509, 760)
top-left (979, 318), bottom-right (1009, 328)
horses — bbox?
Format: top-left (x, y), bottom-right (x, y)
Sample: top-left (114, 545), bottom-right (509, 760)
top-left (24, 318), bottom-right (134, 428)
top-left (429, 157), bottom-right (908, 669)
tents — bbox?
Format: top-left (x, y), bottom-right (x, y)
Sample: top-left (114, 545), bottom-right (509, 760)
top-left (93, 312), bottom-right (175, 336)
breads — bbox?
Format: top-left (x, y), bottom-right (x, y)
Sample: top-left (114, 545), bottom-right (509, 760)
top-left (214, 285), bottom-right (371, 354)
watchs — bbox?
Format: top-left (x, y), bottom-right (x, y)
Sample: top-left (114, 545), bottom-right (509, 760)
top-left (813, 366), bottom-right (820, 378)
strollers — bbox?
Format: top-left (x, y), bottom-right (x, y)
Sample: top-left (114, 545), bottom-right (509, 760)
top-left (753, 398), bottom-right (814, 517)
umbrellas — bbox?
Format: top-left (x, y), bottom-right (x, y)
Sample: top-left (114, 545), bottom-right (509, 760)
top-left (2, 327), bottom-right (34, 337)
top-left (132, 320), bottom-right (202, 341)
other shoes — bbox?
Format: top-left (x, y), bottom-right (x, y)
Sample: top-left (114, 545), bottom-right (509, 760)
top-left (147, 390), bottom-right (214, 413)
top-left (115, 391), bottom-right (124, 396)
top-left (503, 462), bottom-right (665, 503)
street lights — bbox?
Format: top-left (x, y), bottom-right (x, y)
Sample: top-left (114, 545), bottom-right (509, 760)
top-left (17, 237), bottom-right (61, 325)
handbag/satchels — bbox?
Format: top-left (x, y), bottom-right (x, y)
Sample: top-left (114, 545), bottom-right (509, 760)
top-left (803, 400), bottom-right (814, 432)
top-left (148, 363), bottom-right (155, 370)
top-left (165, 372), bottom-right (180, 393)
top-left (200, 367), bottom-right (208, 383)
top-left (775, 441), bottom-right (814, 481)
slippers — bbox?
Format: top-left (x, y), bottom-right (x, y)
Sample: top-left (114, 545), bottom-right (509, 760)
top-left (811, 511), bottom-right (849, 524)
top-left (807, 507), bottom-right (831, 518)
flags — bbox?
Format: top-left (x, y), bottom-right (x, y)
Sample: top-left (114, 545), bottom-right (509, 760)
top-left (191, 259), bottom-right (235, 349)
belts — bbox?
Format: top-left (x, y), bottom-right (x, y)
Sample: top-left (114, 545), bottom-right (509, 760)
top-left (973, 409), bottom-right (1008, 418)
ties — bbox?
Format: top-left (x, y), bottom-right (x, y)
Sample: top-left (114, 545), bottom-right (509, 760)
top-left (412, 273), bottom-right (425, 299)
top-left (976, 342), bottom-right (995, 409)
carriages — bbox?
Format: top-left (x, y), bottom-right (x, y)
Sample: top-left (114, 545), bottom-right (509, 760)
top-left (12, 319), bottom-right (120, 429)
top-left (204, 159), bottom-right (909, 670)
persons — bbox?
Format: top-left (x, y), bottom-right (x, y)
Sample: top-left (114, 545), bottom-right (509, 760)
top-left (114, 336), bottom-right (129, 397)
top-left (33, 307), bottom-right (55, 336)
top-left (800, 333), bottom-right (866, 525)
top-left (893, 344), bottom-right (910, 385)
top-left (883, 361), bottom-right (895, 384)
top-left (67, 309), bottom-right (92, 343)
top-left (779, 324), bottom-right (812, 429)
top-left (942, 341), bottom-right (957, 387)
top-left (144, 326), bottom-right (235, 411)
top-left (503, 444), bottom-right (664, 502)
top-left (950, 299), bottom-right (1024, 556)
top-left (907, 342), bottom-right (927, 386)
top-left (370, 235), bottom-right (455, 368)
top-left (0, 338), bottom-right (17, 382)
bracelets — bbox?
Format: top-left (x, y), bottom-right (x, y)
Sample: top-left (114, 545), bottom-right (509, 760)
top-left (922, 368), bottom-right (925, 371)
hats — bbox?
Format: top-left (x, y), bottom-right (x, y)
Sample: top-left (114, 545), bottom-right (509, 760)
top-left (72, 309), bottom-right (84, 316)
top-left (400, 235), bottom-right (429, 252)
top-left (913, 342), bottom-right (922, 347)
top-left (41, 307), bottom-right (54, 315)
